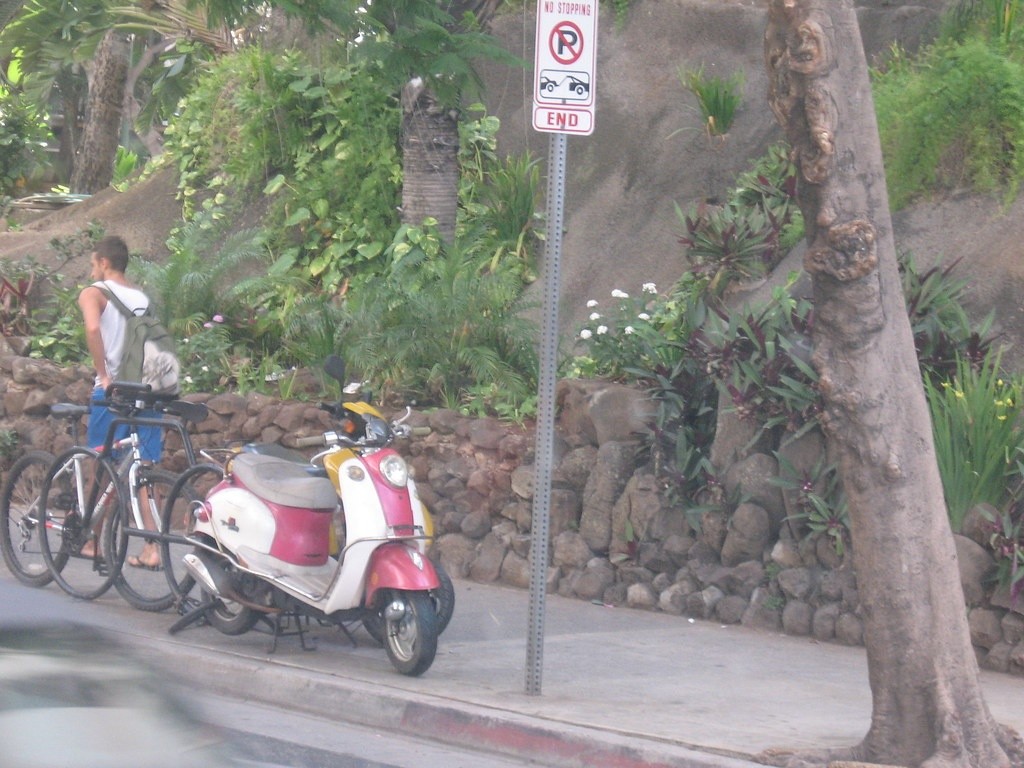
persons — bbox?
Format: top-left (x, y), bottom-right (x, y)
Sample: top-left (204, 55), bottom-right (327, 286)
top-left (71, 236), bottom-right (164, 571)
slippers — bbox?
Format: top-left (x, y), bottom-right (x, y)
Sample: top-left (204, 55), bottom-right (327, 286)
top-left (69, 548), bottom-right (102, 560)
top-left (129, 555), bottom-right (164, 571)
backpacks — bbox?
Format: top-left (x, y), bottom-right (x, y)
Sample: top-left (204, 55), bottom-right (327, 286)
top-left (90, 280), bottom-right (181, 411)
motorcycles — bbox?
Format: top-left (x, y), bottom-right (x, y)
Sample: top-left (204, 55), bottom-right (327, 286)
top-left (0, 354), bottom-right (456, 680)
